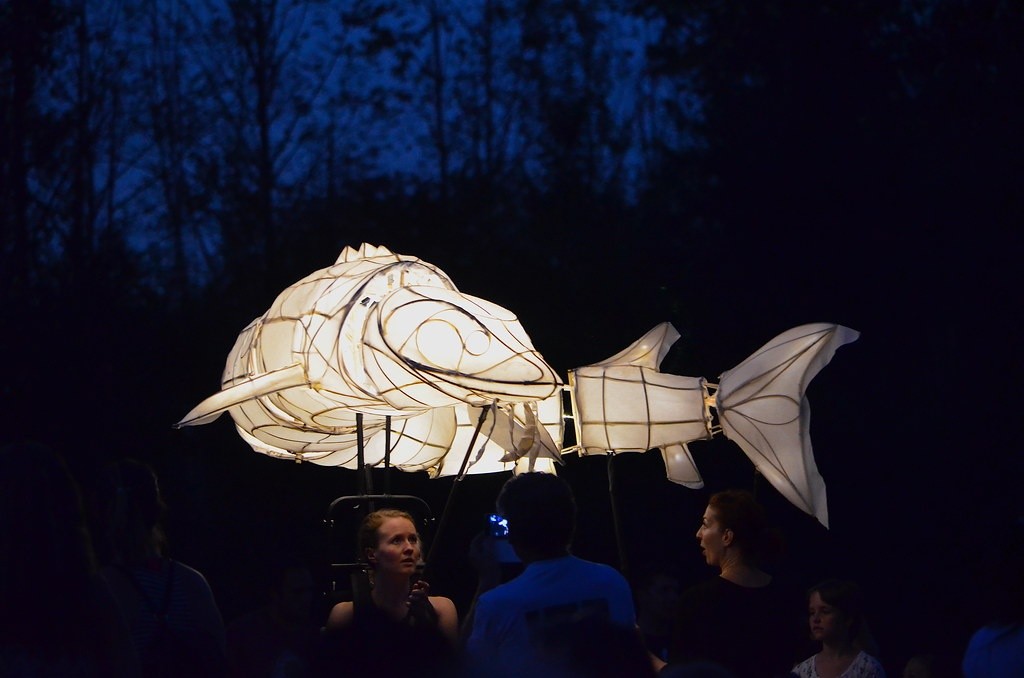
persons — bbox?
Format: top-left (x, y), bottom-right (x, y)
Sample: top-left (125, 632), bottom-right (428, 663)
top-left (902, 505), bottom-right (1024, 678)
top-left (0, 439), bottom-right (226, 678)
top-left (789, 580), bottom-right (885, 678)
top-left (462, 474), bottom-right (636, 678)
top-left (635, 493), bottom-right (788, 678)
top-left (328, 509), bottom-right (459, 678)
